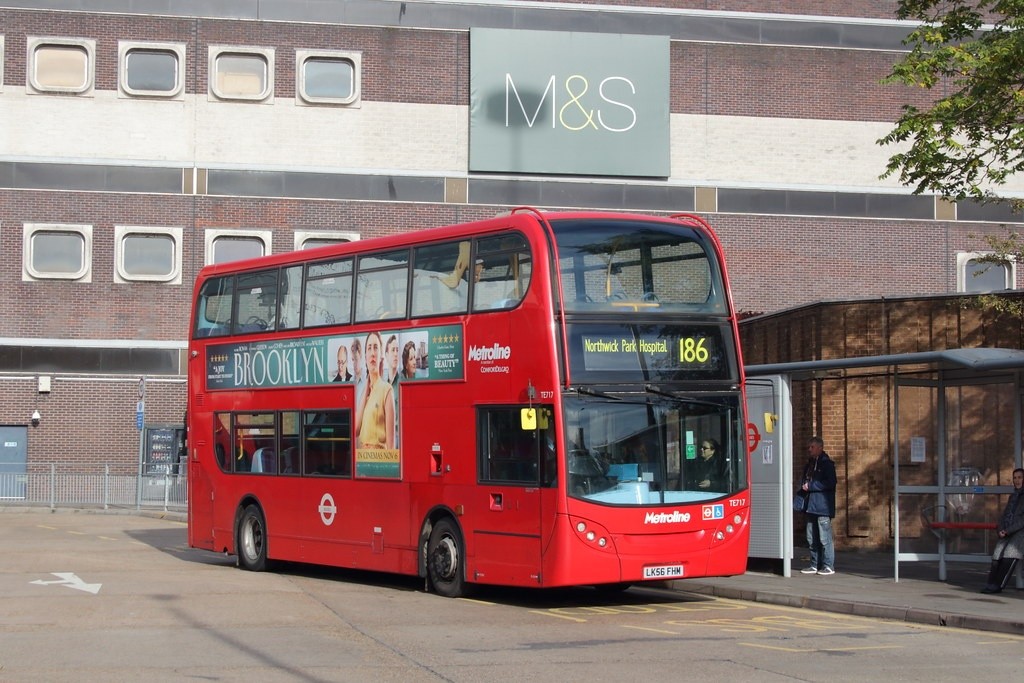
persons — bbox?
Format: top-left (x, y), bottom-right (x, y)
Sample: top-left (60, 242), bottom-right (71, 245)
top-left (980, 468), bottom-right (1024, 593)
top-left (800, 437), bottom-right (837, 575)
top-left (332, 331), bottom-right (416, 450)
top-left (690, 439), bottom-right (723, 491)
top-left (528, 417), bottom-right (577, 479)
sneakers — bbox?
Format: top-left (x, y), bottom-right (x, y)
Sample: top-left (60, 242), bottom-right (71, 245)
top-left (816, 567), bottom-right (835, 575)
top-left (800, 566), bottom-right (817, 574)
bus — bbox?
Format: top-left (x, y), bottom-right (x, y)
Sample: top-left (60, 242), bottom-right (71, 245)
top-left (179, 206), bottom-right (755, 598)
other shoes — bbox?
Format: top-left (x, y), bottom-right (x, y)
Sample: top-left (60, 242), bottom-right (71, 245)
top-left (980, 584), bottom-right (1001, 594)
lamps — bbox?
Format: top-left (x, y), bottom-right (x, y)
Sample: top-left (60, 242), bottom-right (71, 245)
top-left (32, 375), bottom-right (41, 421)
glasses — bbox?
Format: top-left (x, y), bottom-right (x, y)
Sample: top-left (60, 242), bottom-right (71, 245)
top-left (700, 445), bottom-right (712, 450)
top-left (338, 359), bottom-right (347, 365)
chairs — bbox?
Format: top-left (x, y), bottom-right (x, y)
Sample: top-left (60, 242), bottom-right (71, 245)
top-left (251, 447), bottom-right (276, 472)
top-left (572, 291), bottom-right (662, 305)
top-left (221, 316), bottom-right (267, 335)
top-left (281, 447), bottom-right (299, 474)
